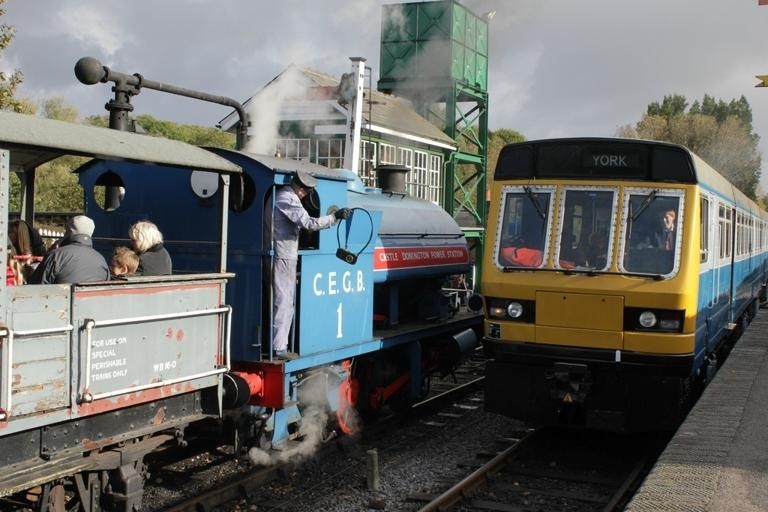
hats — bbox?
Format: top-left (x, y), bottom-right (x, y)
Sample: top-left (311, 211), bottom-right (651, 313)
top-left (294, 169), bottom-right (317, 192)
top-left (66, 215), bottom-right (95, 237)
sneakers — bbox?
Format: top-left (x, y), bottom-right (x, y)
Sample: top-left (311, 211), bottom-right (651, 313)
top-left (273, 352), bottom-right (297, 360)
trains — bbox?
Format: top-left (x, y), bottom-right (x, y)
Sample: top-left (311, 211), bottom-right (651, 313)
top-left (472, 135), bottom-right (768, 411)
top-left (0, 52), bottom-right (484, 511)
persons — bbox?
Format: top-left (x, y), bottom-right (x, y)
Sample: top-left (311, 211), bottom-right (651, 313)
top-left (634, 207), bottom-right (679, 253)
top-left (7, 212), bottom-right (173, 289)
top-left (264, 168), bottom-right (354, 360)
